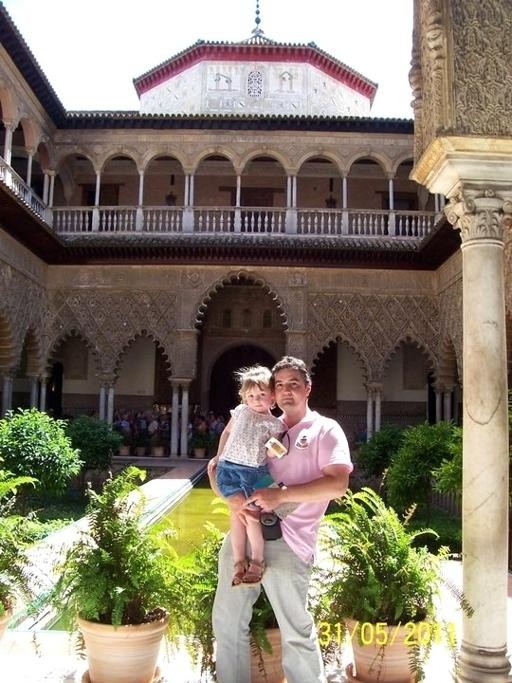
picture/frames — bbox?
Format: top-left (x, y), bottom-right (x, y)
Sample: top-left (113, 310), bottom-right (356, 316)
top-left (150, 444), bottom-right (165, 456)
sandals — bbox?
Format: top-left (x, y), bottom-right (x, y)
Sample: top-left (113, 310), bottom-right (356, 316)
top-left (242, 560), bottom-right (265, 583)
top-left (231, 560), bottom-right (247, 585)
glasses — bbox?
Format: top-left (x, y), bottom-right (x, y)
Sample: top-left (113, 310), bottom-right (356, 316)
top-left (281, 431), bottom-right (290, 456)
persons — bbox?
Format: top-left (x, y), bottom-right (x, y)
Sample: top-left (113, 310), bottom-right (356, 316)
top-left (208, 364), bottom-right (289, 590)
top-left (112, 408), bottom-right (225, 459)
top-left (212, 355), bottom-right (354, 682)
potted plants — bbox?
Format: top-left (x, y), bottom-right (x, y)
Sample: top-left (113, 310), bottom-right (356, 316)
top-left (174, 496), bottom-right (288, 683)
top-left (322, 484), bottom-right (440, 681)
top-left (60, 464), bottom-right (172, 683)
top-left (0, 474), bottom-right (40, 639)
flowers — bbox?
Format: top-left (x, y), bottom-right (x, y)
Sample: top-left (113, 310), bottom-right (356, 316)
top-left (132, 409), bottom-right (148, 444)
top-left (188, 418), bottom-right (210, 447)
top-left (149, 406), bottom-right (169, 442)
top-left (114, 409), bottom-right (131, 439)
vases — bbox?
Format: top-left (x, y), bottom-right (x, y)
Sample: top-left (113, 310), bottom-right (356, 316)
top-left (194, 448), bottom-right (206, 457)
top-left (119, 445), bottom-right (130, 454)
top-left (137, 446), bottom-right (146, 454)
